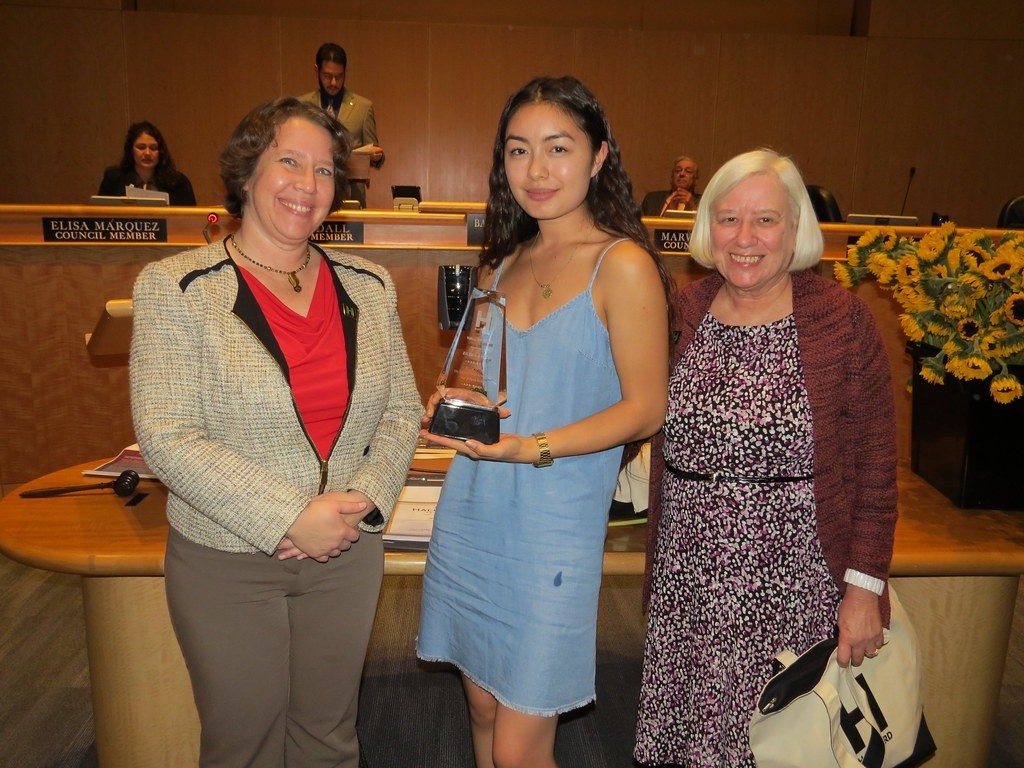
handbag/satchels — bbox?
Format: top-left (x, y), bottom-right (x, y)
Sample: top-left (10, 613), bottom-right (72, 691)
top-left (746, 577), bottom-right (925, 767)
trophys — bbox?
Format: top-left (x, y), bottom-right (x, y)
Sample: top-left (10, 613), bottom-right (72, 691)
top-left (428, 287), bottom-right (506, 445)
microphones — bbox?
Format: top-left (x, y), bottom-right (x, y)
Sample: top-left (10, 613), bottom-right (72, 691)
top-left (901, 167), bottom-right (915, 216)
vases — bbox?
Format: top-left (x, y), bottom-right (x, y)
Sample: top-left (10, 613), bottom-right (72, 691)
top-left (907, 341), bottom-right (1024, 510)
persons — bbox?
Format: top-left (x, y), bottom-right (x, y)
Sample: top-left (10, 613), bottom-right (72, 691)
top-left (295, 42), bottom-right (385, 209)
top-left (98, 122), bottom-right (198, 208)
top-left (132, 96), bottom-right (427, 768)
top-left (612, 150), bottom-right (897, 768)
top-left (416, 74), bottom-right (669, 768)
top-left (640, 156), bottom-right (702, 216)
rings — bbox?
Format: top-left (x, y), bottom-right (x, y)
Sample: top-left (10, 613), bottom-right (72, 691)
top-left (865, 649), bottom-right (880, 658)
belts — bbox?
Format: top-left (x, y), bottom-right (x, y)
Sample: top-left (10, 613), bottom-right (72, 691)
top-left (664, 455), bottom-right (814, 485)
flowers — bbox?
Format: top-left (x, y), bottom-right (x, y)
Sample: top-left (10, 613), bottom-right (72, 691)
top-left (831, 222), bottom-right (1024, 404)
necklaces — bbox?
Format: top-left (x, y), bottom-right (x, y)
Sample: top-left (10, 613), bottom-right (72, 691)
top-left (231, 232), bottom-right (310, 292)
top-left (530, 225), bottom-right (596, 298)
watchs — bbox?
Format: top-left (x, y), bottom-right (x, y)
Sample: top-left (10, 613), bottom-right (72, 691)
top-left (531, 432), bottom-right (554, 468)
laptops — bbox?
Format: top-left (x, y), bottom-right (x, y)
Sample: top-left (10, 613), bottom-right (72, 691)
top-left (90, 195), bottom-right (168, 206)
top-left (341, 200), bottom-right (361, 210)
top-left (846, 213), bottom-right (919, 226)
top-left (664, 210), bottom-right (696, 219)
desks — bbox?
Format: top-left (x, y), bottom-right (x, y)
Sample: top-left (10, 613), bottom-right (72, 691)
top-left (0, 428), bottom-right (1024, 768)
